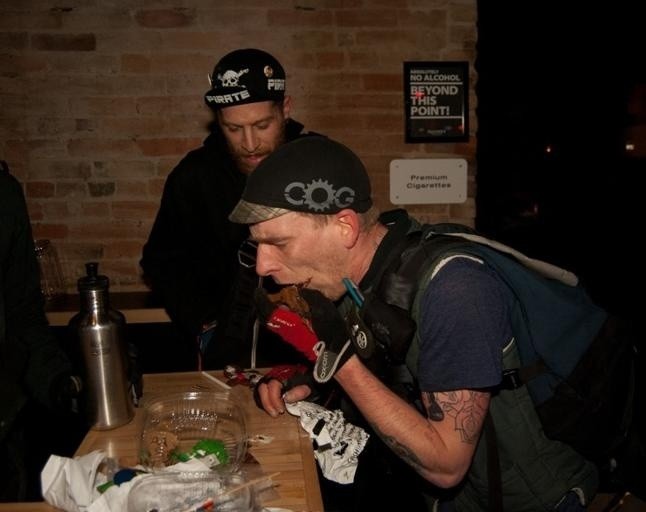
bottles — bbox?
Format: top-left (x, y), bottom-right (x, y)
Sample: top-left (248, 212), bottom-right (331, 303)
top-left (67, 261), bottom-right (144, 432)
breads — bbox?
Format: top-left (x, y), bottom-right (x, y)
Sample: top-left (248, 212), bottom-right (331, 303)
top-left (264, 284), bottom-right (311, 317)
top-left (148, 430), bottom-right (179, 466)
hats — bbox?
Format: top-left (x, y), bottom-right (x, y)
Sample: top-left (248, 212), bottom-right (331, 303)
top-left (204, 47), bottom-right (286, 108)
top-left (227, 136), bottom-right (372, 225)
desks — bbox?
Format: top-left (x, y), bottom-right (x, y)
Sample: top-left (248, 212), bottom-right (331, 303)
top-left (45, 366), bottom-right (326, 512)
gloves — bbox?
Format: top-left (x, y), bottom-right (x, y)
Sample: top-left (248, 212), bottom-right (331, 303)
top-left (268, 291), bottom-right (354, 383)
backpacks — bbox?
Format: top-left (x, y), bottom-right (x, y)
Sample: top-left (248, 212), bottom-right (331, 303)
top-left (372, 222), bottom-right (646, 490)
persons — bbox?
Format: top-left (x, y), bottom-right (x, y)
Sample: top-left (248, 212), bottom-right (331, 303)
top-left (138, 48), bottom-right (328, 372)
top-left (0, 157), bottom-right (80, 505)
top-left (228, 132), bottom-right (606, 512)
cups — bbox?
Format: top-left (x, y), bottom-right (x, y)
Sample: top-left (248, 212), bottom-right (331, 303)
top-left (33, 238), bottom-right (66, 301)
